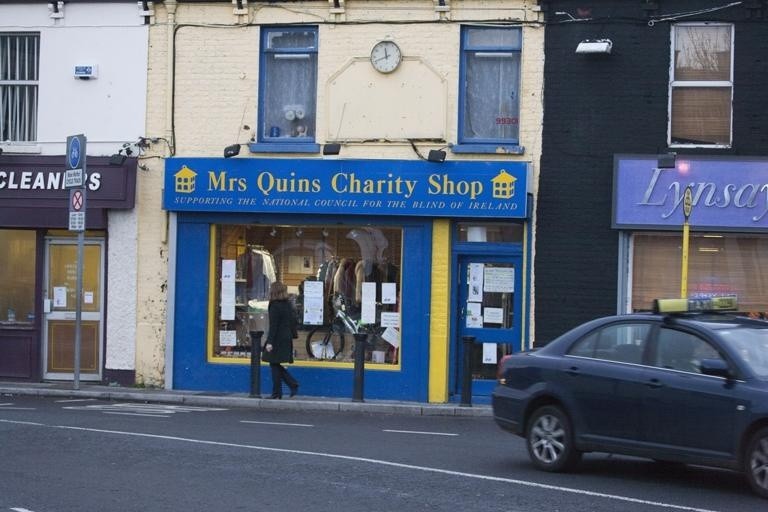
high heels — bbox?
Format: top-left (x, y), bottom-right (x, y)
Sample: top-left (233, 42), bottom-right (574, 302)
top-left (288, 381), bottom-right (299, 397)
top-left (265, 393), bottom-right (281, 399)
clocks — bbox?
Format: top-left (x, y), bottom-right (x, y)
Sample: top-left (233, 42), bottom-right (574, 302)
top-left (368, 40), bottom-right (403, 75)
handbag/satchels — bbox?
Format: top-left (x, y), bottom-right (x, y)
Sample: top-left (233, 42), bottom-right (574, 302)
top-left (290, 318), bottom-right (297, 338)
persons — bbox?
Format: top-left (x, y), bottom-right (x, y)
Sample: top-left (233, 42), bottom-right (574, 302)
top-left (259, 281), bottom-right (300, 399)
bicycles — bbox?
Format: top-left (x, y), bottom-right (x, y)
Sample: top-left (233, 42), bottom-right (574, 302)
top-left (304, 284), bottom-right (390, 360)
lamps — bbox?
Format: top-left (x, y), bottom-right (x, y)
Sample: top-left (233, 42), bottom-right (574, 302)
top-left (269, 224), bottom-right (330, 239)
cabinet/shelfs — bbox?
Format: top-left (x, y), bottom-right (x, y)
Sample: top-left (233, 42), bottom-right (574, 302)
top-left (218, 277), bottom-right (247, 308)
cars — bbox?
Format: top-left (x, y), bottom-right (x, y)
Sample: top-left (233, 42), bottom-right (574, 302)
top-left (492, 293), bottom-right (768, 496)
